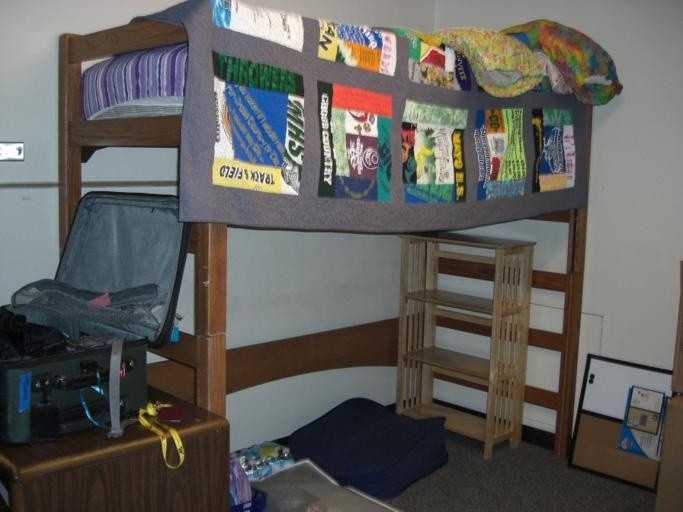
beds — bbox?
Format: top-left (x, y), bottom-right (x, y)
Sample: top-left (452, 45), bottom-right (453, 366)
top-left (56, 1), bottom-right (595, 462)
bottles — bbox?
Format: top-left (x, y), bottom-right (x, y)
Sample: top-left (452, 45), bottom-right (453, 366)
top-left (229, 440), bottom-right (295, 484)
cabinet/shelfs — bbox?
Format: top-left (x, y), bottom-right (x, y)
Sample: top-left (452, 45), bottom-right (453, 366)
top-left (392, 226), bottom-right (539, 461)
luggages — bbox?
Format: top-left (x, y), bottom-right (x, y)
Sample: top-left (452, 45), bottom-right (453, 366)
top-left (2, 191), bottom-right (190, 445)
